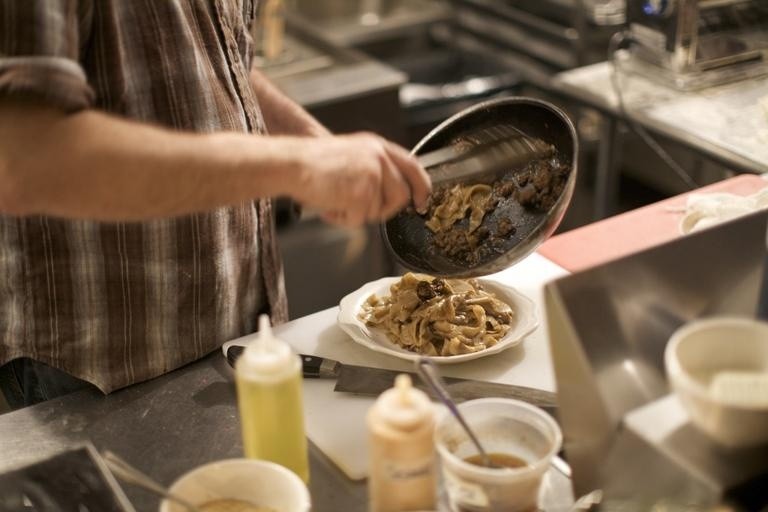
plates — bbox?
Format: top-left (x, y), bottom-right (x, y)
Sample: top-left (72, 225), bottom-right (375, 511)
top-left (337, 276), bottom-right (542, 366)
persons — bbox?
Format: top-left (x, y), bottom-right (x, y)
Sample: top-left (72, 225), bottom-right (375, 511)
top-left (0, 0), bottom-right (435, 410)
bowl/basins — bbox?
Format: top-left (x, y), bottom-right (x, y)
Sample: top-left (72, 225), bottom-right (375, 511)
top-left (158, 458), bottom-right (312, 512)
top-left (663, 316), bottom-right (768, 449)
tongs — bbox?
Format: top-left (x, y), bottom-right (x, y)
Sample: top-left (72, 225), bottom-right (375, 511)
top-left (415, 123), bottom-right (555, 190)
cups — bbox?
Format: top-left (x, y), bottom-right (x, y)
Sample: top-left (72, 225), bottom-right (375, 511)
top-left (433, 397), bottom-right (563, 512)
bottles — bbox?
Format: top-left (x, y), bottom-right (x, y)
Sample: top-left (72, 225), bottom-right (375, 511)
top-left (234, 313), bottom-right (309, 487)
top-left (366, 374), bottom-right (438, 512)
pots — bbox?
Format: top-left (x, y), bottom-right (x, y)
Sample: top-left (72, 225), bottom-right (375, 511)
top-left (381, 96), bottom-right (579, 278)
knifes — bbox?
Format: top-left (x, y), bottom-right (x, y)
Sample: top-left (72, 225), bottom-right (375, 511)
top-left (225, 345), bottom-right (568, 408)
